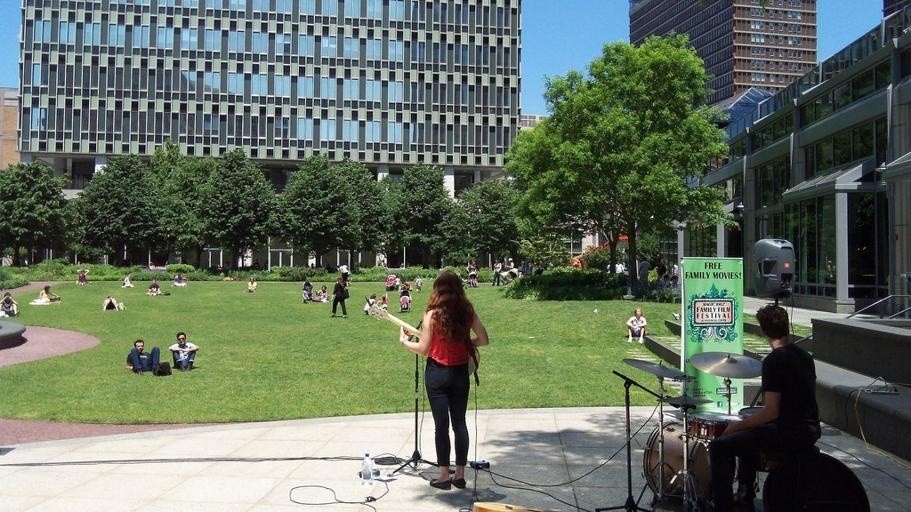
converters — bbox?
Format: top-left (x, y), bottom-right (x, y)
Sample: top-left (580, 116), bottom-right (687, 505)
top-left (470, 461), bottom-right (490, 469)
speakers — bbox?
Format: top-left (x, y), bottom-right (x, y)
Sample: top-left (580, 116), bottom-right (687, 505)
top-left (753, 239), bottom-right (796, 298)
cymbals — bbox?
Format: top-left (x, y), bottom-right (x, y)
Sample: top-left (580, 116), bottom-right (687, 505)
top-left (622, 358), bottom-right (686, 380)
top-left (690, 352), bottom-right (764, 379)
top-left (363, 302), bottom-right (481, 378)
top-left (657, 396), bottom-right (713, 405)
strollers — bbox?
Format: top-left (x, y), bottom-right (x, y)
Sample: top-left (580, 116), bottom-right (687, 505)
top-left (385, 274), bottom-right (397, 291)
top-left (469, 273), bottom-right (478, 287)
top-left (400, 291), bottom-right (411, 312)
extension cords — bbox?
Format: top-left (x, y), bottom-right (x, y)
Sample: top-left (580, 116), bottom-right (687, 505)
top-left (865, 388), bottom-right (900, 394)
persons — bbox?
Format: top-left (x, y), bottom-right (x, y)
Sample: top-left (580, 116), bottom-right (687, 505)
top-left (247, 276), bottom-right (257, 292)
top-left (102, 295), bottom-right (118, 312)
top-left (39, 285), bottom-right (61, 303)
top-left (169, 333), bottom-right (199, 371)
top-left (625, 308), bottom-right (647, 344)
top-left (707, 305), bottom-right (822, 512)
top-left (76, 269), bottom-right (89, 286)
top-left (606, 258), bottom-right (678, 289)
top-left (121, 274), bottom-right (134, 288)
top-left (174, 273), bottom-right (187, 287)
top-left (399, 270), bottom-right (489, 489)
top-left (363, 258), bottom-right (518, 315)
top-left (301, 264), bottom-right (350, 318)
top-left (0, 292), bottom-right (18, 315)
top-left (126, 339), bottom-right (161, 375)
top-left (145, 279), bottom-right (162, 296)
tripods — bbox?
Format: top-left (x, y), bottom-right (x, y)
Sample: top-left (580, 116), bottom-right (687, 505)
top-left (595, 380), bottom-right (656, 512)
top-left (388, 335), bottom-right (457, 474)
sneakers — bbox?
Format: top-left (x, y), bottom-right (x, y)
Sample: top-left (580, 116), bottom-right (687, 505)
top-left (452, 477), bottom-right (466, 489)
top-left (430, 479), bottom-right (452, 490)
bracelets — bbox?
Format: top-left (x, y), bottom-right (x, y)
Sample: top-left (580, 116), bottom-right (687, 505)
top-left (401, 339), bottom-right (406, 346)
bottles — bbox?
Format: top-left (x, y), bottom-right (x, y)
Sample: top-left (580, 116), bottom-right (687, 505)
top-left (360, 453), bottom-right (373, 494)
top-left (179, 350), bottom-right (183, 358)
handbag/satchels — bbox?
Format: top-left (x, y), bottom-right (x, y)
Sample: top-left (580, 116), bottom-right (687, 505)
top-left (337, 289), bottom-right (350, 300)
top-left (153, 362), bottom-right (171, 376)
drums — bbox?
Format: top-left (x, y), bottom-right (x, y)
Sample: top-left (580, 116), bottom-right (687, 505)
top-left (643, 422), bottom-right (711, 502)
top-left (738, 406), bottom-right (761, 417)
top-left (685, 411), bottom-right (742, 438)
